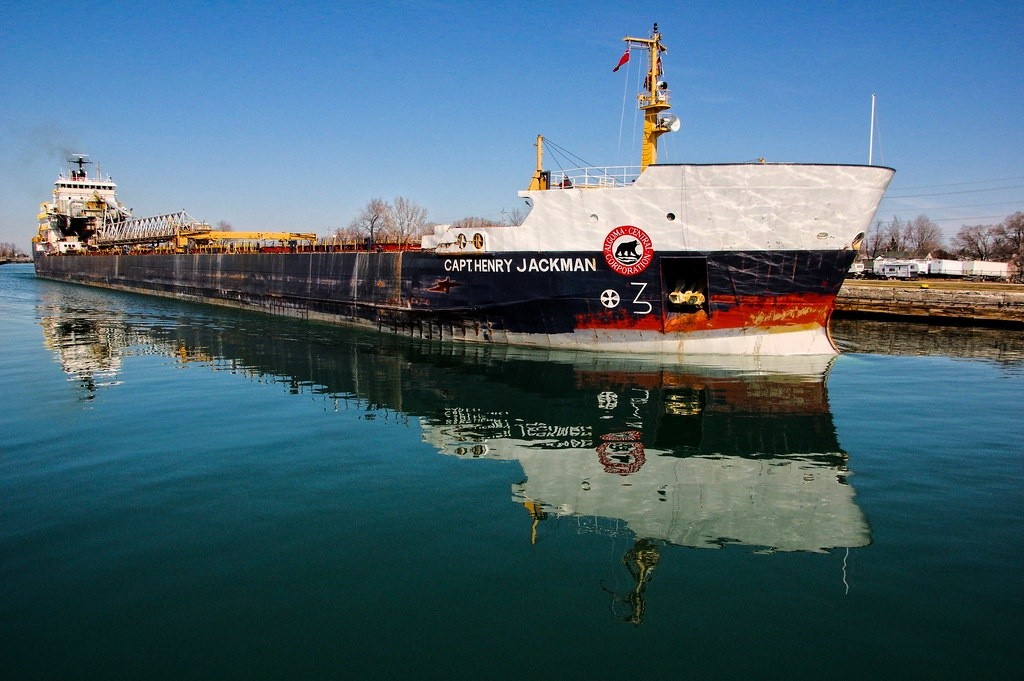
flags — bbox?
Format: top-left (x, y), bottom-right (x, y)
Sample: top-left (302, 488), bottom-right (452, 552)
top-left (612, 49), bottom-right (629, 72)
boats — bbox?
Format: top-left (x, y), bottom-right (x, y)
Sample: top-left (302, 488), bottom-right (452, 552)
top-left (32, 24), bottom-right (894, 383)
